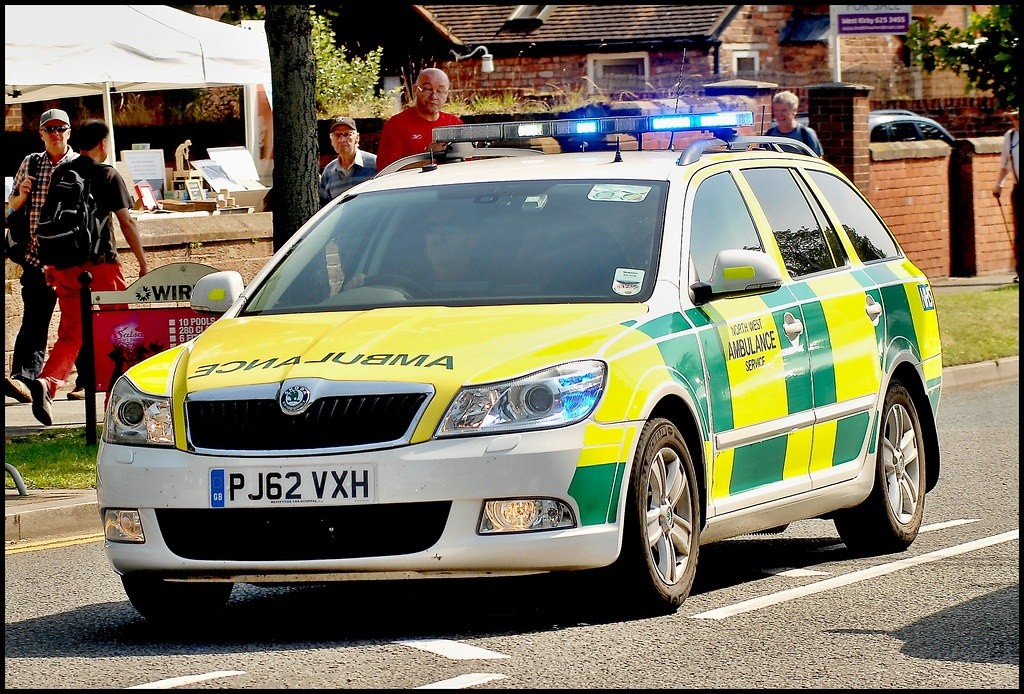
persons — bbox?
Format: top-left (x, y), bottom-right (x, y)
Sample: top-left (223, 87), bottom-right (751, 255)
top-left (759, 90), bottom-right (824, 161)
top-left (413, 203), bottom-right (479, 295)
top-left (993, 107), bottom-right (1019, 284)
top-left (320, 116), bottom-right (378, 275)
top-left (30, 118), bottom-right (147, 427)
top-left (4, 109), bottom-right (86, 402)
top-left (377, 69), bottom-right (473, 173)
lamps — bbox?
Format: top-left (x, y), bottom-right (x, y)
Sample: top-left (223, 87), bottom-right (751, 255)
top-left (450, 46), bottom-right (494, 73)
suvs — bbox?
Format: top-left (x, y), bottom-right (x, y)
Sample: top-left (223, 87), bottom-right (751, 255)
top-left (763, 109), bottom-right (959, 145)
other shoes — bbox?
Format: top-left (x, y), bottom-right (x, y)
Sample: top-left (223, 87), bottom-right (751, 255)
top-left (1014, 276), bottom-right (1019, 283)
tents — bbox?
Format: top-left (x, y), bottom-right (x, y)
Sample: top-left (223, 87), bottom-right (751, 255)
top-left (5, 5), bottom-right (263, 171)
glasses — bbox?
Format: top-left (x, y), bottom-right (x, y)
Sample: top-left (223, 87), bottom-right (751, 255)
top-left (415, 84), bottom-right (450, 99)
top-left (330, 132), bottom-right (356, 140)
top-left (40, 126), bottom-right (70, 133)
top-left (425, 223), bottom-right (469, 238)
top-left (1010, 115), bottom-right (1019, 119)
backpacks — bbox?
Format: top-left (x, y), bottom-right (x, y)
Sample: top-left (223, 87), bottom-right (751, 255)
top-left (36, 162), bottom-right (114, 271)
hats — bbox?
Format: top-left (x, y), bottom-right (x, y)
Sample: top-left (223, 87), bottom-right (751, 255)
top-left (1003, 106), bottom-right (1020, 115)
top-left (40, 109), bottom-right (71, 127)
top-left (330, 117), bottom-right (356, 132)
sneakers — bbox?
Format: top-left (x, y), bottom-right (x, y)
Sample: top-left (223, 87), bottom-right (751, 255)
top-left (67, 387), bottom-right (85, 399)
top-left (30, 378), bottom-right (53, 426)
top-left (4, 375), bottom-right (33, 403)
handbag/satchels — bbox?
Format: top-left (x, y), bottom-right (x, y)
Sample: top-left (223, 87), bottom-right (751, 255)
top-left (5, 152), bottom-right (39, 263)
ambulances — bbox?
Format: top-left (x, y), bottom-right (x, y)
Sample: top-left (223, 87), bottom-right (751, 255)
top-left (92, 112), bottom-right (942, 619)
